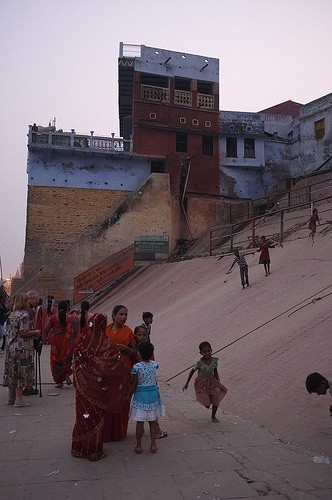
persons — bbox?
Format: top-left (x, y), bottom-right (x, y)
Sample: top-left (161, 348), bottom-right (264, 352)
top-left (70, 313), bottom-right (131, 462)
top-left (305, 372), bottom-right (332, 416)
top-left (139, 312), bottom-right (154, 344)
top-left (104, 304), bottom-right (137, 440)
top-left (134, 326), bottom-right (167, 439)
top-left (2, 293), bottom-right (41, 407)
top-left (22, 290), bottom-right (39, 396)
top-left (256, 236), bottom-right (279, 276)
top-left (43, 301), bottom-right (74, 388)
top-left (228, 249), bottom-right (255, 290)
top-left (1, 293), bottom-right (79, 374)
top-left (75, 300), bottom-right (95, 341)
top-left (183, 341), bottom-right (227, 423)
top-left (127, 342), bottom-right (166, 455)
top-left (302, 208), bottom-right (321, 243)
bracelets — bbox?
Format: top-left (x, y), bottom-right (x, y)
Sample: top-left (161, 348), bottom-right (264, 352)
top-left (125, 347), bottom-right (131, 353)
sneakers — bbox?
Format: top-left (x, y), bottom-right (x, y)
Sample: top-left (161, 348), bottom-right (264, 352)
top-left (13, 399), bottom-right (30, 408)
top-left (6, 398), bottom-right (14, 405)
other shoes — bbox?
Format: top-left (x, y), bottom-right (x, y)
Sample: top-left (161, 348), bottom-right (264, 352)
top-left (154, 432), bottom-right (168, 439)
top-left (150, 447), bottom-right (158, 453)
top-left (247, 284), bottom-right (249, 287)
top-left (55, 383), bottom-right (63, 388)
top-left (242, 286), bottom-right (245, 290)
top-left (265, 272), bottom-right (271, 277)
top-left (211, 416), bottom-right (219, 423)
top-left (135, 446), bottom-right (142, 454)
top-left (65, 374), bottom-right (71, 386)
top-left (22, 388), bottom-right (39, 396)
top-left (205, 405), bottom-right (210, 409)
